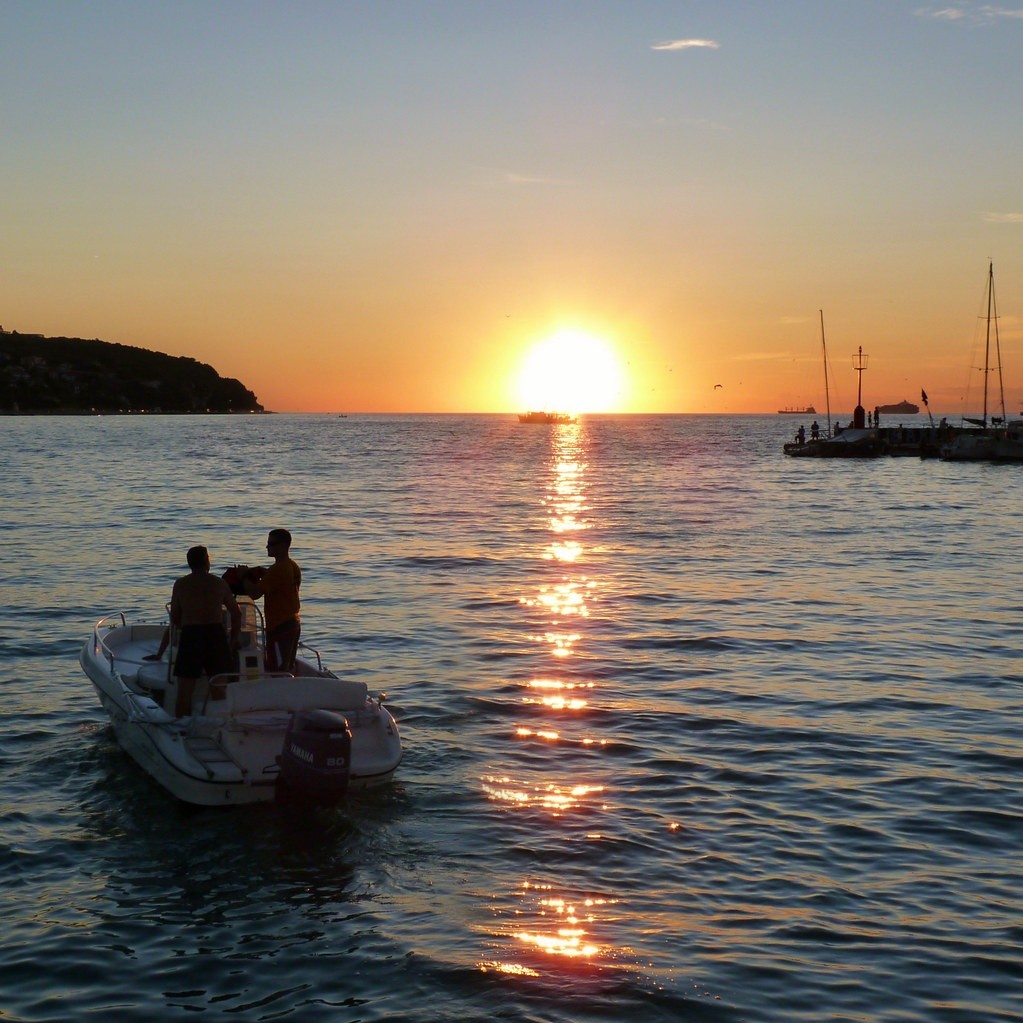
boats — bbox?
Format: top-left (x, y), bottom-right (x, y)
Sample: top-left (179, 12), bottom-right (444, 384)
top-left (338, 413), bottom-right (348, 418)
top-left (517, 411), bottom-right (577, 425)
top-left (778, 406), bottom-right (817, 414)
top-left (79, 592), bottom-right (403, 806)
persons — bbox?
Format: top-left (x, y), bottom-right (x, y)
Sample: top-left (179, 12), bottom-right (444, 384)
top-left (897, 424), bottom-right (904, 444)
top-left (794, 425), bottom-right (805, 445)
top-left (834, 421), bottom-right (840, 437)
top-left (848, 421), bottom-right (853, 429)
top-left (242, 528), bottom-right (304, 681)
top-left (873, 407), bottom-right (879, 428)
top-left (811, 421), bottom-right (820, 440)
top-left (142, 611), bottom-right (242, 684)
top-left (868, 411), bottom-right (873, 429)
top-left (939, 417), bottom-right (948, 432)
top-left (169, 544), bottom-right (245, 721)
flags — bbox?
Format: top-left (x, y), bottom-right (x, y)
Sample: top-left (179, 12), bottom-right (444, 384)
top-left (921, 389), bottom-right (929, 406)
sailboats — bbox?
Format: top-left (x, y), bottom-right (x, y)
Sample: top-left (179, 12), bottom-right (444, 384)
top-left (783, 252), bottom-right (1022, 466)
